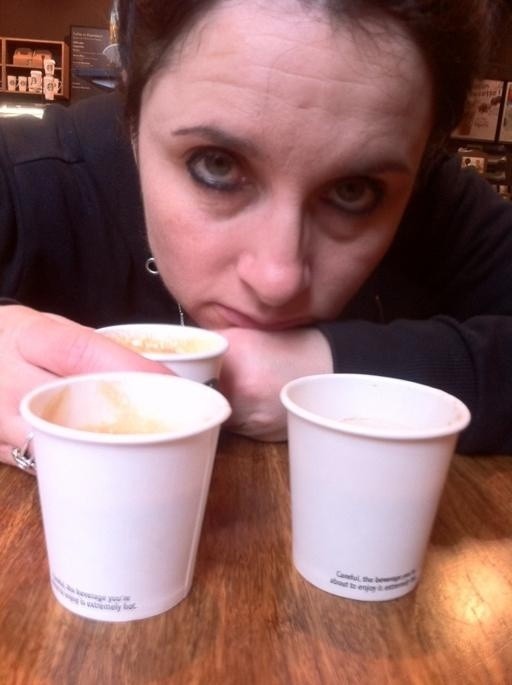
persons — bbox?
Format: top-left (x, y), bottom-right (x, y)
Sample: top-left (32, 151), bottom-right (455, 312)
top-left (0, 0), bottom-right (512, 478)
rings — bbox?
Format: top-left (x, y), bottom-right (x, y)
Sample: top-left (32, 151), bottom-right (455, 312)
top-left (14, 436), bottom-right (37, 476)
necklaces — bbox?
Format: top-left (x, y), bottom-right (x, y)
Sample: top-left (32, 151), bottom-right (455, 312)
top-left (177, 303), bottom-right (184, 325)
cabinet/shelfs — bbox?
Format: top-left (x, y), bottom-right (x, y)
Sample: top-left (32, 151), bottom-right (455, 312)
top-left (0, 36), bottom-right (71, 101)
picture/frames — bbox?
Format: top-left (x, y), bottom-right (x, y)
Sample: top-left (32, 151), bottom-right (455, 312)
top-left (69, 25), bottom-right (122, 78)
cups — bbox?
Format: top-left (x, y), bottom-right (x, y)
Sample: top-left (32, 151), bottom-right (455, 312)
top-left (95, 323), bottom-right (229, 394)
top-left (280, 371), bottom-right (471, 602)
top-left (7, 58), bottom-right (63, 102)
top-left (21, 369), bottom-right (232, 621)
